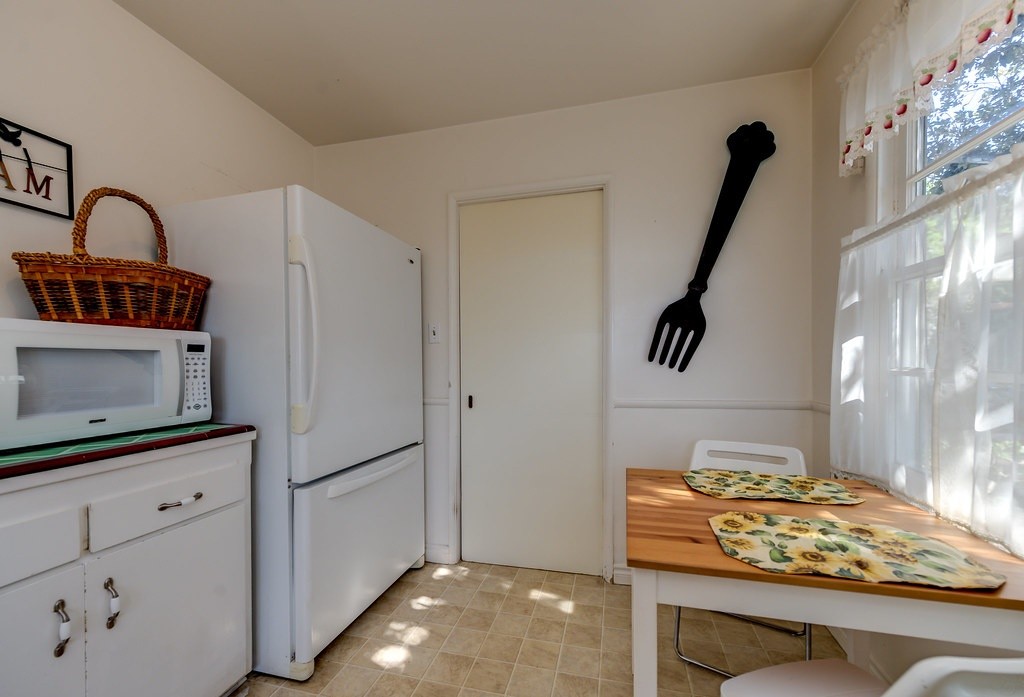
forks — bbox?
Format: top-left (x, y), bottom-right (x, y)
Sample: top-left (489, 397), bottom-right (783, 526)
top-left (648, 122), bottom-right (778, 373)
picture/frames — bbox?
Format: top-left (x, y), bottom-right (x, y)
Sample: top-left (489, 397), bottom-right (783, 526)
top-left (0, 117), bottom-right (74, 220)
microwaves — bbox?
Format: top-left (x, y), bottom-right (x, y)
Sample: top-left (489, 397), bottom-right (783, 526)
top-left (0, 318), bottom-right (211, 453)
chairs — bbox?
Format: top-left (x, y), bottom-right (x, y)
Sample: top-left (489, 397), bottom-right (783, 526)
top-left (720, 655), bottom-right (1024, 696)
top-left (672, 440), bottom-right (810, 678)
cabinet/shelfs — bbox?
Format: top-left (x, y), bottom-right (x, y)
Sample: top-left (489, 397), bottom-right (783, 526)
top-left (0, 426), bottom-right (256, 697)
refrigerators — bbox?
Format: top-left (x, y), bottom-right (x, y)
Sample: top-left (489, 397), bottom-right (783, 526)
top-left (170, 185), bottom-right (422, 681)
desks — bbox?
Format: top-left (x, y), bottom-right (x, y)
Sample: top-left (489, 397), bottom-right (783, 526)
top-left (625, 467), bottom-right (1024, 697)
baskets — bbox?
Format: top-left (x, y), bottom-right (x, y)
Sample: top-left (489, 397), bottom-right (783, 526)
top-left (12, 188), bottom-right (211, 331)
